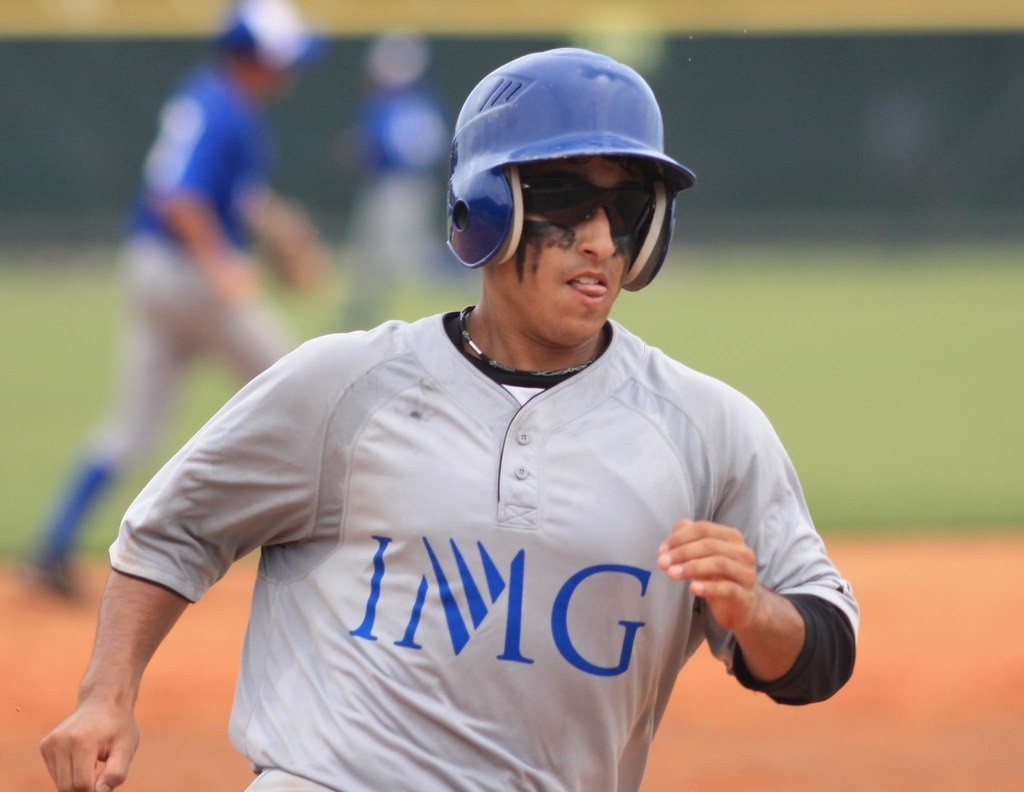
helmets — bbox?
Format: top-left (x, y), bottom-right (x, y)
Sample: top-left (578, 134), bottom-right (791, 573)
top-left (446, 47), bottom-right (697, 292)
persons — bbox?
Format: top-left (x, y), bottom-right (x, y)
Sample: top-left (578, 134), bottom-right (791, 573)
top-left (39, 42), bottom-right (862, 792)
top-left (339, 35), bottom-right (448, 326)
top-left (17, 0), bottom-right (335, 604)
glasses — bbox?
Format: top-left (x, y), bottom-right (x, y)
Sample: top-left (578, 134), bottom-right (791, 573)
top-left (520, 169), bottom-right (655, 249)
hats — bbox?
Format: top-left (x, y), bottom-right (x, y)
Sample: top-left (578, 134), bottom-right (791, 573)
top-left (219, 4), bottom-right (327, 71)
